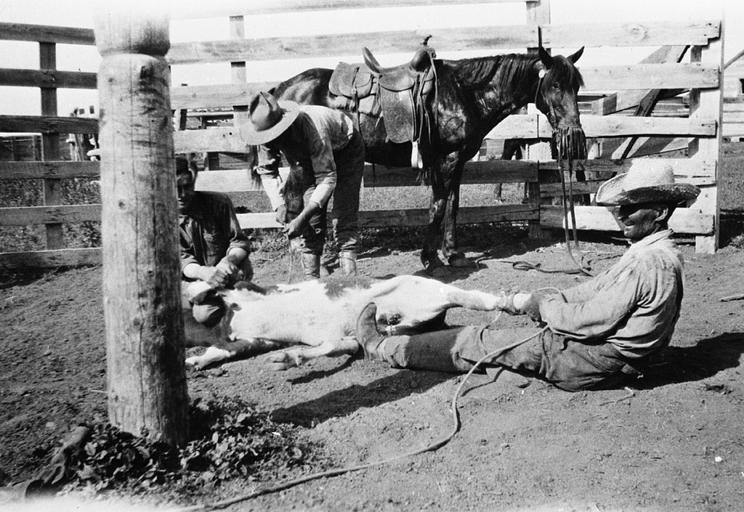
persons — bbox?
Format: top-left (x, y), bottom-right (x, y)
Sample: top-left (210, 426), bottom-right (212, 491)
top-left (239, 89), bottom-right (367, 279)
top-left (174, 155), bottom-right (255, 326)
top-left (352, 158), bottom-right (702, 392)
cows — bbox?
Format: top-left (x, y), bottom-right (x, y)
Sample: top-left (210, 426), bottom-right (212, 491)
top-left (181, 278), bottom-right (535, 373)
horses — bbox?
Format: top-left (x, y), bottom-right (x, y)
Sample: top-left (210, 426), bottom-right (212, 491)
top-left (250, 46), bottom-right (588, 276)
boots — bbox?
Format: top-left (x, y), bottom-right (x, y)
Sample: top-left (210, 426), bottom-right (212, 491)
top-left (354, 301), bottom-right (390, 364)
top-left (329, 216), bottom-right (361, 278)
top-left (298, 231), bottom-right (322, 282)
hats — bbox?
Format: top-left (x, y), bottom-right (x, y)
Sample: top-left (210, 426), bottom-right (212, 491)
top-left (593, 157), bottom-right (702, 209)
top-left (238, 90), bottom-right (302, 147)
